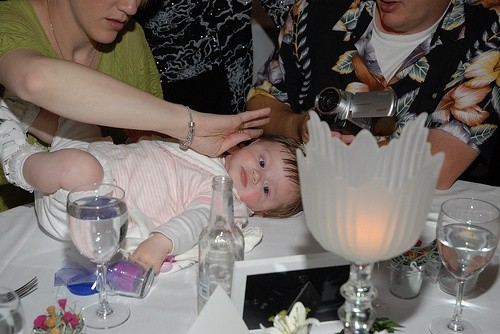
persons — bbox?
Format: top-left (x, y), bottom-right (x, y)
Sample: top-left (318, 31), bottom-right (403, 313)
top-left (96, 0), bottom-right (299, 146)
top-left (0, 86), bottom-right (306, 275)
top-left (0, 0), bottom-right (271, 213)
top-left (245, 0), bottom-right (500, 190)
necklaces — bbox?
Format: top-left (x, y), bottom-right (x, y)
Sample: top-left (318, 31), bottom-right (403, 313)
top-left (47, 0), bottom-right (97, 68)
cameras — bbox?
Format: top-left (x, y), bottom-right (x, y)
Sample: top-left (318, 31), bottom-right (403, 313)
top-left (314, 87), bottom-right (397, 136)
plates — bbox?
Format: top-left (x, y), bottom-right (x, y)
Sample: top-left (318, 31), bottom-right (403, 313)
top-left (412, 211), bottom-right (440, 250)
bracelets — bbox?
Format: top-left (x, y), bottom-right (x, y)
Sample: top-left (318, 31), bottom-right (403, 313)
top-left (178, 105), bottom-right (195, 151)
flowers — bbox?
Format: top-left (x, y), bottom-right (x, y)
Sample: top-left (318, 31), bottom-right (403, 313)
top-left (259, 302), bottom-right (321, 334)
top-left (33, 298), bottom-right (84, 334)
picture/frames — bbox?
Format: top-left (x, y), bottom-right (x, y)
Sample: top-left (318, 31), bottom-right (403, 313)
top-left (231, 252), bottom-right (350, 331)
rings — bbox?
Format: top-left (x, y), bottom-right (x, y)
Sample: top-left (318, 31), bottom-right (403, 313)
top-left (240, 123), bottom-right (244, 129)
top-left (240, 129), bottom-right (244, 133)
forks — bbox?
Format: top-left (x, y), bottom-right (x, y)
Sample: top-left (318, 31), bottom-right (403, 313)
top-left (13, 276), bottom-right (38, 298)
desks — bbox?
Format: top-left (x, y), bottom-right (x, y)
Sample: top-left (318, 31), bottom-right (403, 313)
top-left (0, 180), bottom-right (500, 334)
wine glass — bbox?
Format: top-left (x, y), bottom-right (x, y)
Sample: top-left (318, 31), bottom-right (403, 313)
top-left (68, 183), bottom-right (131, 328)
top-left (428, 197), bottom-right (500, 334)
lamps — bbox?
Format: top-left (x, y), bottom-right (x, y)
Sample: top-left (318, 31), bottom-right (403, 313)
top-left (295, 110), bottom-right (446, 334)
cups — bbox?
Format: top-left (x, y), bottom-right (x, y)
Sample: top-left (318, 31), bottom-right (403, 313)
top-left (0, 286), bottom-right (26, 333)
top-left (102, 249), bottom-right (153, 298)
top-left (389, 258), bottom-right (426, 299)
top-left (439, 263), bottom-right (482, 298)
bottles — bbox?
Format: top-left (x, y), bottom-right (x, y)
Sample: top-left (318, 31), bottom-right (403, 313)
top-left (196, 176), bottom-right (245, 316)
